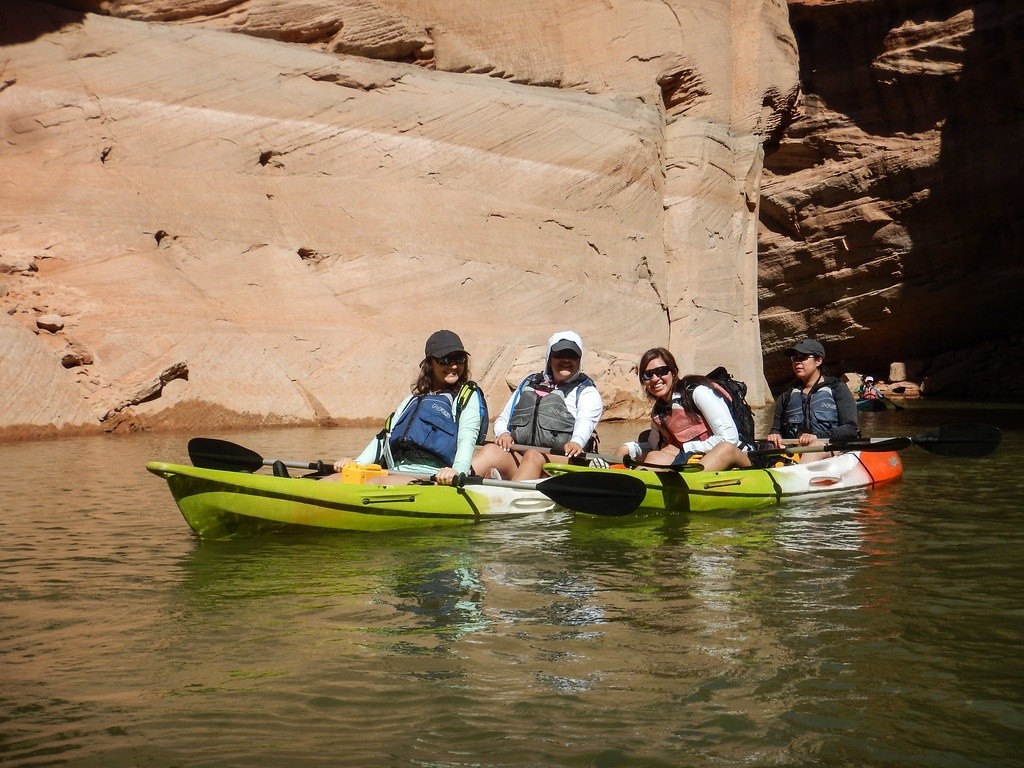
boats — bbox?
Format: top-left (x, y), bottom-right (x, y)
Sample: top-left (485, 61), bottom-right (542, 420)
top-left (543, 451), bottom-right (903, 512)
top-left (854, 399), bottom-right (888, 412)
top-left (146, 461), bottom-right (559, 539)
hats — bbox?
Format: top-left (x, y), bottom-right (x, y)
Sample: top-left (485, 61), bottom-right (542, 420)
top-left (552, 339), bottom-right (582, 356)
top-left (865, 376), bottom-right (873, 382)
top-left (424, 329), bottom-right (471, 358)
top-left (785, 339), bottom-right (825, 360)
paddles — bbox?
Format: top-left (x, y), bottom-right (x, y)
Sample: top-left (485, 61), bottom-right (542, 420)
top-left (511, 442), bottom-right (705, 474)
top-left (186, 436), bottom-right (647, 518)
top-left (741, 435), bottom-right (912, 456)
top-left (854, 371), bottom-right (903, 411)
top-left (781, 426), bottom-right (1003, 457)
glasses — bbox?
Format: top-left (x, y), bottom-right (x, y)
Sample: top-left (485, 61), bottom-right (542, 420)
top-left (552, 350), bottom-right (579, 360)
top-left (433, 355), bottom-right (466, 365)
top-left (791, 354), bottom-right (815, 362)
top-left (642, 365), bottom-right (671, 380)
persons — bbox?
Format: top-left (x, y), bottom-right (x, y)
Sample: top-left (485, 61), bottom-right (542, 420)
top-left (467, 330), bottom-right (603, 482)
top-left (697, 339), bottom-right (859, 471)
top-left (240, 330), bottom-right (480, 486)
top-left (854, 375), bottom-right (883, 402)
top-left (588, 347), bottom-right (754, 470)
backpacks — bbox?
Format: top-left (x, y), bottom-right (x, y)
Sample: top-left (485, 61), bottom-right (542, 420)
top-left (685, 366), bottom-right (757, 451)
